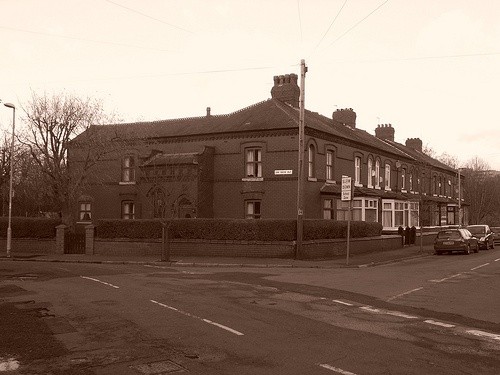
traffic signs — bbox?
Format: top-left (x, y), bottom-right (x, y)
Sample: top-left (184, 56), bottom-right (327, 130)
top-left (341, 175), bottom-right (351, 201)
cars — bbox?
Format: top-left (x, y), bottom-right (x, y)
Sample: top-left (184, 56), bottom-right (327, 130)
top-left (434, 229), bottom-right (480, 255)
top-left (489, 226), bottom-right (500, 246)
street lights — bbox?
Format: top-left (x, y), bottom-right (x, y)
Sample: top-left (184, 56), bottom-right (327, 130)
top-left (3, 102), bottom-right (16, 257)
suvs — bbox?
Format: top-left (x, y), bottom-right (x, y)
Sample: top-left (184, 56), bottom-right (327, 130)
top-left (466, 224), bottom-right (495, 250)
